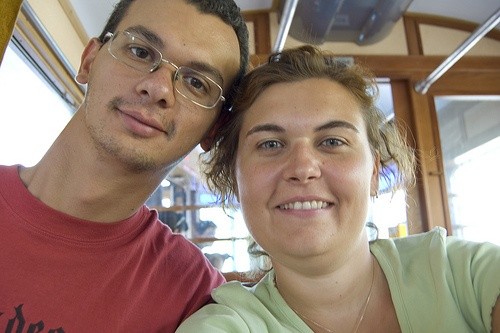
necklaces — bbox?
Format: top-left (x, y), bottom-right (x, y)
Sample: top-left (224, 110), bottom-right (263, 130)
top-left (277, 259), bottom-right (377, 331)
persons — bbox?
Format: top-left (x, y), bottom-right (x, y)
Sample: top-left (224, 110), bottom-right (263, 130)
top-left (0, 0), bottom-right (252, 330)
top-left (158, 209), bottom-right (188, 237)
top-left (170, 44), bottom-right (498, 332)
top-left (194, 218), bottom-right (231, 271)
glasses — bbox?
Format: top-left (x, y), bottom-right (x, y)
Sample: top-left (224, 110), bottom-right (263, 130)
top-left (102, 31), bottom-right (226, 108)
